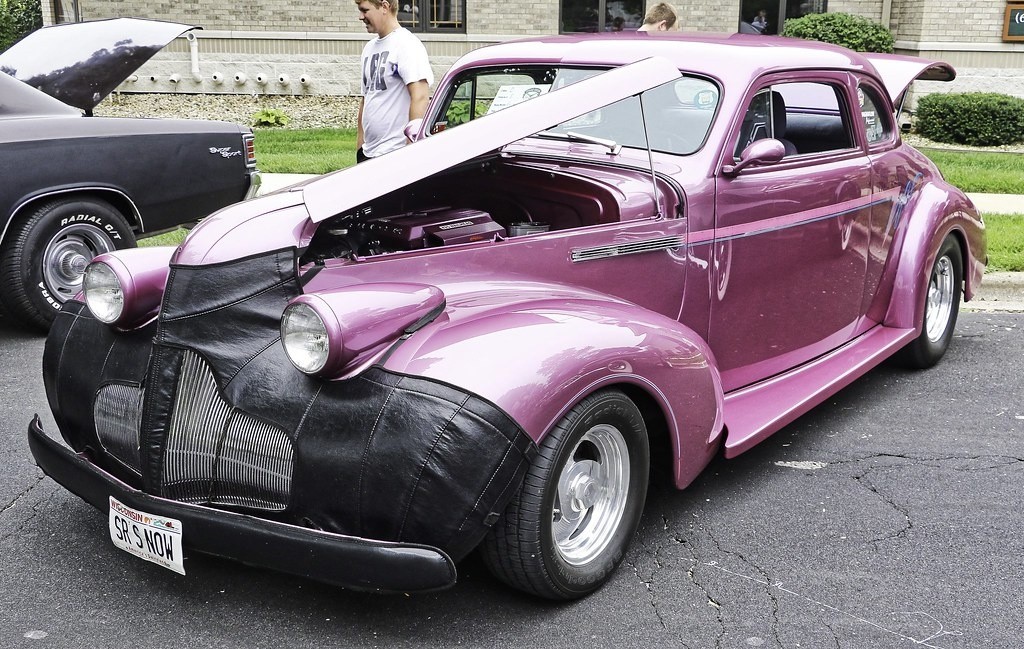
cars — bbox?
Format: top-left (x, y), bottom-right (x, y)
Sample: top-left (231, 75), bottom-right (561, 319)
top-left (0, 17), bottom-right (261, 336)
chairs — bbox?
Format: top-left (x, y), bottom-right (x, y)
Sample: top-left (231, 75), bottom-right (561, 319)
top-left (735, 90), bottom-right (797, 162)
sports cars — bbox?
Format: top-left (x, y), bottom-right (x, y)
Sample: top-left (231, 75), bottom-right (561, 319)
top-left (28, 31), bottom-right (988, 606)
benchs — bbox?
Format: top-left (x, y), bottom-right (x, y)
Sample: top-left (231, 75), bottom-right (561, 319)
top-left (783, 113), bottom-right (852, 154)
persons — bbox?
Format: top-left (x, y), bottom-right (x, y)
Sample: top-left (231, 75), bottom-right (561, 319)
top-left (354, 0), bottom-right (434, 165)
top-left (636, 2), bottom-right (679, 33)
top-left (752, 9), bottom-right (766, 30)
